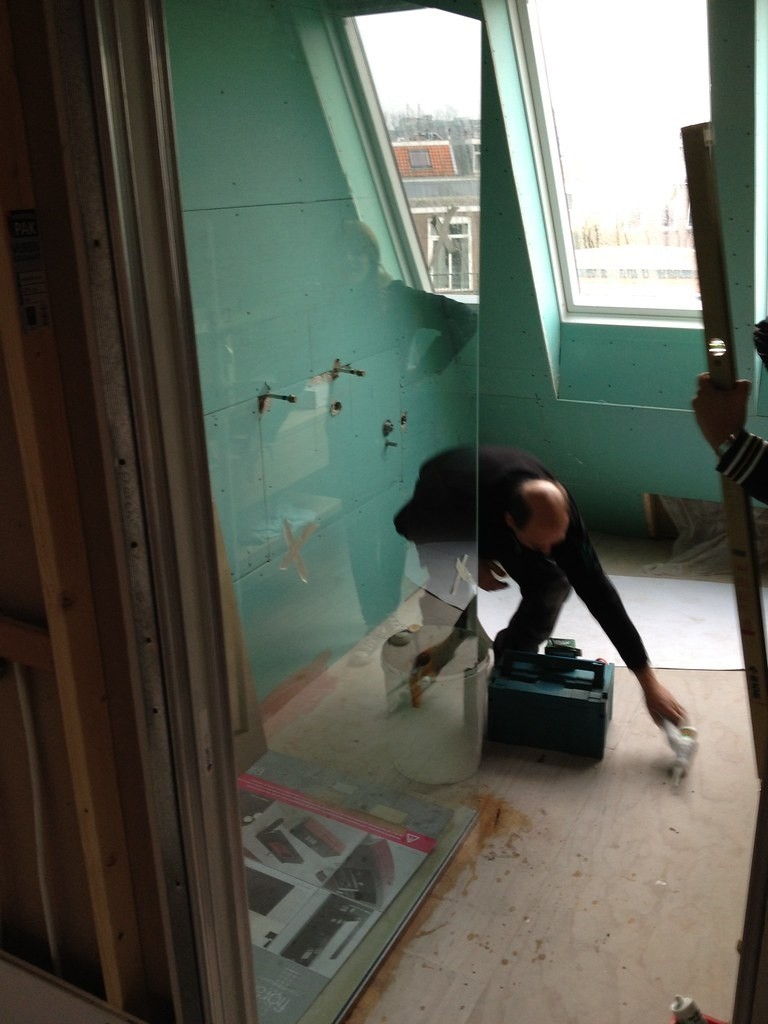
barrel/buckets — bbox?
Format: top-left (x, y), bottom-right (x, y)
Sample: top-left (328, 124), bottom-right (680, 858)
top-left (381, 626), bottom-right (491, 786)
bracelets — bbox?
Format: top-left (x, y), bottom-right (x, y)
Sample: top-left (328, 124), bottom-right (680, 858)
top-left (717, 430), bottom-right (739, 457)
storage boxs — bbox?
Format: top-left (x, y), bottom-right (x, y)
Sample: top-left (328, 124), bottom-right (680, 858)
top-left (486, 647), bottom-right (614, 762)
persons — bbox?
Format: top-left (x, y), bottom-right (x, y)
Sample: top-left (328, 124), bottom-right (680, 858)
top-left (322, 217), bottom-right (479, 631)
top-left (691, 317), bottom-right (768, 505)
top-left (394, 415), bottom-right (691, 730)
